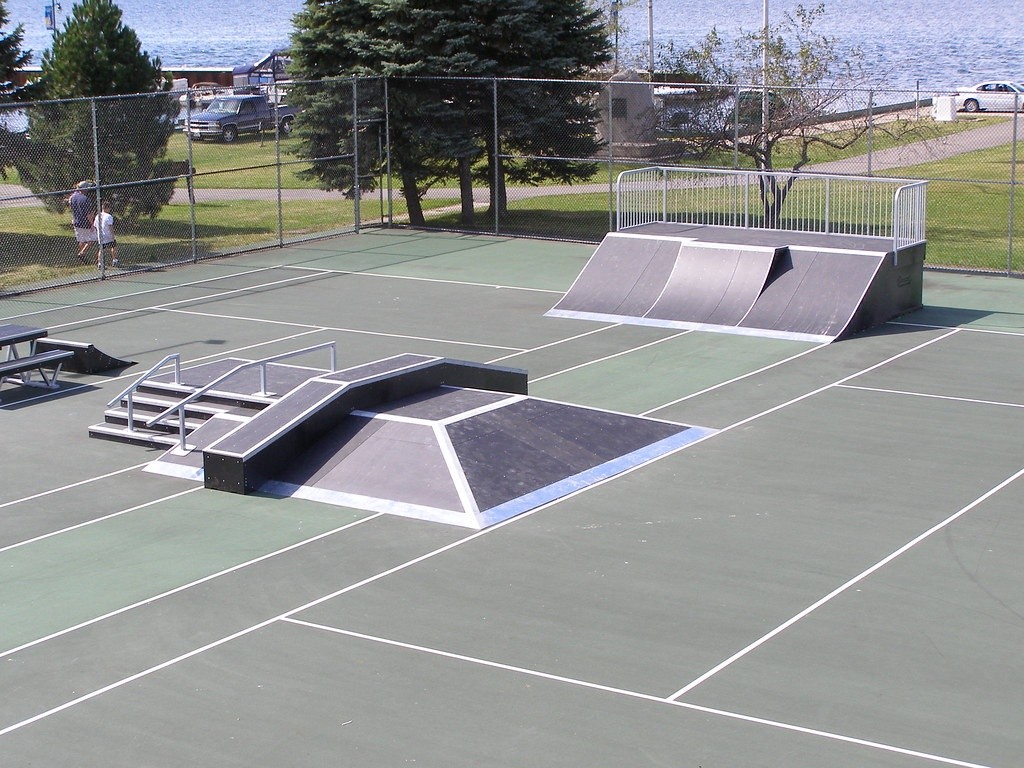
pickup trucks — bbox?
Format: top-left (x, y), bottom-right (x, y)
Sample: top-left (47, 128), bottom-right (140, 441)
top-left (182, 95), bottom-right (303, 142)
top-left (233, 85), bottom-right (261, 95)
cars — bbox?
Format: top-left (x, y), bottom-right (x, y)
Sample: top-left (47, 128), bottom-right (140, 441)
top-left (257, 86), bottom-right (270, 93)
top-left (953, 80), bottom-right (1024, 113)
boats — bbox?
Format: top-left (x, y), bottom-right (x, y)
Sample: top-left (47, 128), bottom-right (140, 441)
top-left (179, 85), bottom-right (225, 108)
top-left (725, 88), bottom-right (787, 128)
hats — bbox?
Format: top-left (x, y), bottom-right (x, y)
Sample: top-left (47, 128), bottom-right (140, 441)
top-left (79, 181), bottom-right (93, 189)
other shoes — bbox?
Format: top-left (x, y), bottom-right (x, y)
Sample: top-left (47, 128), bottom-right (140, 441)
top-left (97, 264), bottom-right (107, 270)
top-left (112, 261), bottom-right (123, 268)
top-left (77, 253), bottom-right (88, 259)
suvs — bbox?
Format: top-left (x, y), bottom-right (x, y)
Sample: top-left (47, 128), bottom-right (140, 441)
top-left (270, 81), bottom-right (295, 102)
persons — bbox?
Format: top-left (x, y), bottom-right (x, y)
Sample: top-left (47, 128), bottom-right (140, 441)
top-left (68, 179), bottom-right (98, 264)
top-left (93, 201), bottom-right (123, 270)
